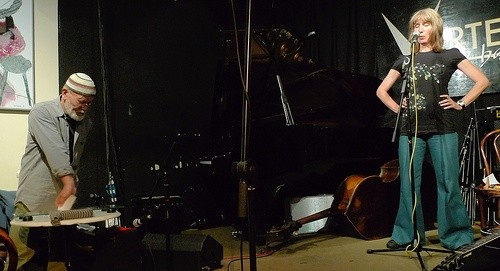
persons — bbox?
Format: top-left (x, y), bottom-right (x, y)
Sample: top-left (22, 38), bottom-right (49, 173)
top-left (4, 72), bottom-right (96, 271)
top-left (376, 8), bottom-right (491, 251)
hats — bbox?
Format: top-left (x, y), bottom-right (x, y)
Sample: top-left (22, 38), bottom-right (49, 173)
top-left (66, 72), bottom-right (96, 96)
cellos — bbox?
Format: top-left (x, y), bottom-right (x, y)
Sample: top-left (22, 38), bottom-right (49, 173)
top-left (263, 157), bottom-right (402, 242)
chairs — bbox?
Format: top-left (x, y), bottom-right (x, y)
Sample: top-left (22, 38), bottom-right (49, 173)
top-left (473, 129), bottom-right (500, 230)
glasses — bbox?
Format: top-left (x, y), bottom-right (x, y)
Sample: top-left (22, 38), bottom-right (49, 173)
top-left (71, 91), bottom-right (96, 108)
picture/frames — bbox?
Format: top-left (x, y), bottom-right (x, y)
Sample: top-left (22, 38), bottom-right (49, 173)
top-left (0, 0), bottom-right (36, 111)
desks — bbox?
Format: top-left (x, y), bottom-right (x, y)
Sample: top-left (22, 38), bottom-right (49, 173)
top-left (11, 210), bottom-right (121, 271)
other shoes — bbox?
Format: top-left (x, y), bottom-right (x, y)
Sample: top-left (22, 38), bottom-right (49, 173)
top-left (387, 239), bottom-right (398, 248)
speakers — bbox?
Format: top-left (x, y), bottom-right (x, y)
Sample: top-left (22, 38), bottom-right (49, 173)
top-left (138, 233), bottom-right (223, 271)
top-left (432, 231), bottom-right (500, 271)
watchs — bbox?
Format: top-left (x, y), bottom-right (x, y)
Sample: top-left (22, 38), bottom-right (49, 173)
top-left (456, 99), bottom-right (466, 108)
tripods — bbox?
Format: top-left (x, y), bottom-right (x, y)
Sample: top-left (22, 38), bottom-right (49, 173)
top-left (366, 44), bottom-right (455, 271)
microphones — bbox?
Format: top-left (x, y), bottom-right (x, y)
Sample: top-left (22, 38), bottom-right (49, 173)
top-left (132, 214), bottom-right (152, 228)
top-left (411, 31), bottom-right (421, 45)
